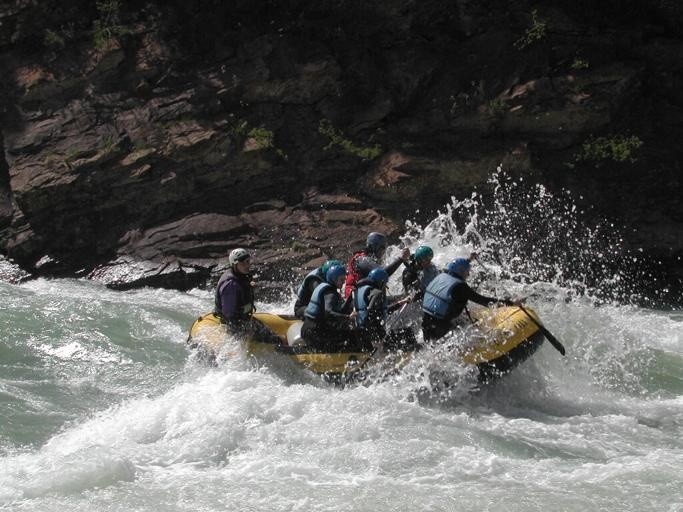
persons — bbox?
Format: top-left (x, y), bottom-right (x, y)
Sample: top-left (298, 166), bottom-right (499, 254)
top-left (300, 264), bottom-right (359, 350)
top-left (402, 245), bottom-right (482, 302)
top-left (213, 248), bottom-right (285, 348)
top-left (293, 259), bottom-right (345, 320)
top-left (421, 256), bottom-right (523, 344)
top-left (343, 231), bottom-right (411, 305)
top-left (350, 266), bottom-right (412, 352)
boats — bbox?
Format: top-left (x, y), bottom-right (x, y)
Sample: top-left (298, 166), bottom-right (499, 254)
top-left (186, 299), bottom-right (546, 394)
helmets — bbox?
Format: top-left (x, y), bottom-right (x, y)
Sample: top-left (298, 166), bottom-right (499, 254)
top-left (322, 260), bottom-right (347, 281)
top-left (228, 248), bottom-right (250, 267)
top-left (449, 259), bottom-right (470, 274)
top-left (368, 268), bottom-right (390, 282)
top-left (366, 232), bottom-right (385, 251)
top-left (413, 246), bottom-right (433, 259)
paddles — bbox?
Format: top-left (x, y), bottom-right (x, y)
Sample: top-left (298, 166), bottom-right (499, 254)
top-left (351, 290), bottom-right (361, 343)
top-left (340, 301), bottom-right (409, 389)
top-left (474, 255), bottom-right (565, 356)
